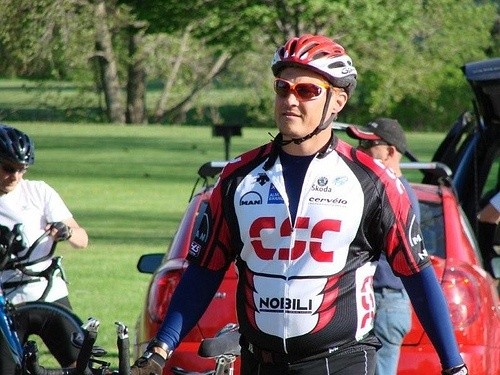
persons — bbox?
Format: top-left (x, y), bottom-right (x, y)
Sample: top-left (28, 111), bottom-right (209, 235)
top-left (0, 127), bottom-right (94, 375)
top-left (477, 191), bottom-right (500, 224)
top-left (342, 115), bottom-right (422, 375)
top-left (127, 35), bottom-right (468, 375)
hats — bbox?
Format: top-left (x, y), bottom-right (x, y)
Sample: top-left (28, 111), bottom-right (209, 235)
top-left (345, 118), bottom-right (408, 155)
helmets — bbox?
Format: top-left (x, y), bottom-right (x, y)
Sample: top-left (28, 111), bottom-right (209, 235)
top-left (270, 34), bottom-right (358, 98)
top-left (0, 125), bottom-right (35, 164)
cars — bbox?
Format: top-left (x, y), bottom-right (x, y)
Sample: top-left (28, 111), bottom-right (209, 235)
top-left (135, 157), bottom-right (500, 375)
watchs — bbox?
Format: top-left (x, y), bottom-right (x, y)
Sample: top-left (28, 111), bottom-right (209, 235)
top-left (67, 226), bottom-right (73, 241)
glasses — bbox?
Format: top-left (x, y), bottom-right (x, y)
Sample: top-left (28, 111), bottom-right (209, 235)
top-left (272, 77), bottom-right (334, 101)
top-left (1, 164), bottom-right (28, 175)
top-left (358, 139), bottom-right (387, 150)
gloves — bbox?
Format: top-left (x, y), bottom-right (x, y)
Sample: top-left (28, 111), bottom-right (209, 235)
top-left (129, 351), bottom-right (166, 375)
